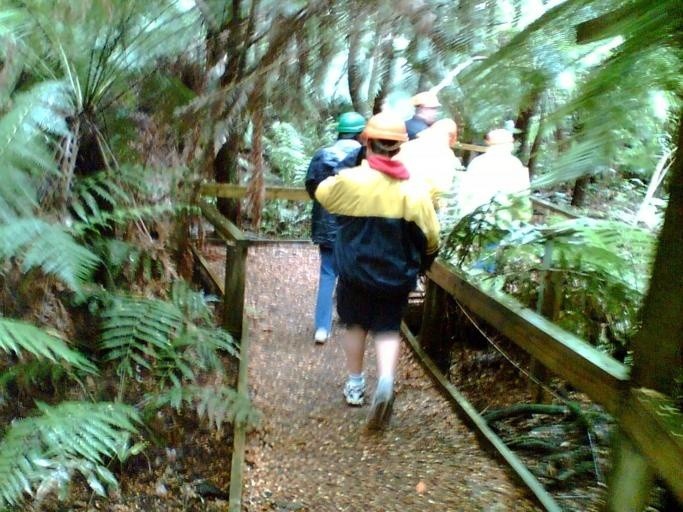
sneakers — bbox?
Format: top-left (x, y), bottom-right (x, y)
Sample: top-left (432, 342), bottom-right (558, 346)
top-left (315, 328), bottom-right (330, 342)
top-left (366, 381), bottom-right (395, 430)
top-left (344, 381), bottom-right (367, 406)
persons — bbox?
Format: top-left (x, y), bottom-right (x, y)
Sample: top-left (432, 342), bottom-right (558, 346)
top-left (405, 92), bottom-right (442, 140)
top-left (303, 111), bottom-right (367, 346)
top-left (465, 128), bottom-right (533, 222)
top-left (395, 119), bottom-right (458, 218)
top-left (313, 110), bottom-right (440, 434)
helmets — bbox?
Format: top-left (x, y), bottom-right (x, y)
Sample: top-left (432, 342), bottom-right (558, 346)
top-left (336, 111), bottom-right (367, 134)
top-left (411, 91), bottom-right (441, 108)
top-left (366, 112), bottom-right (409, 143)
